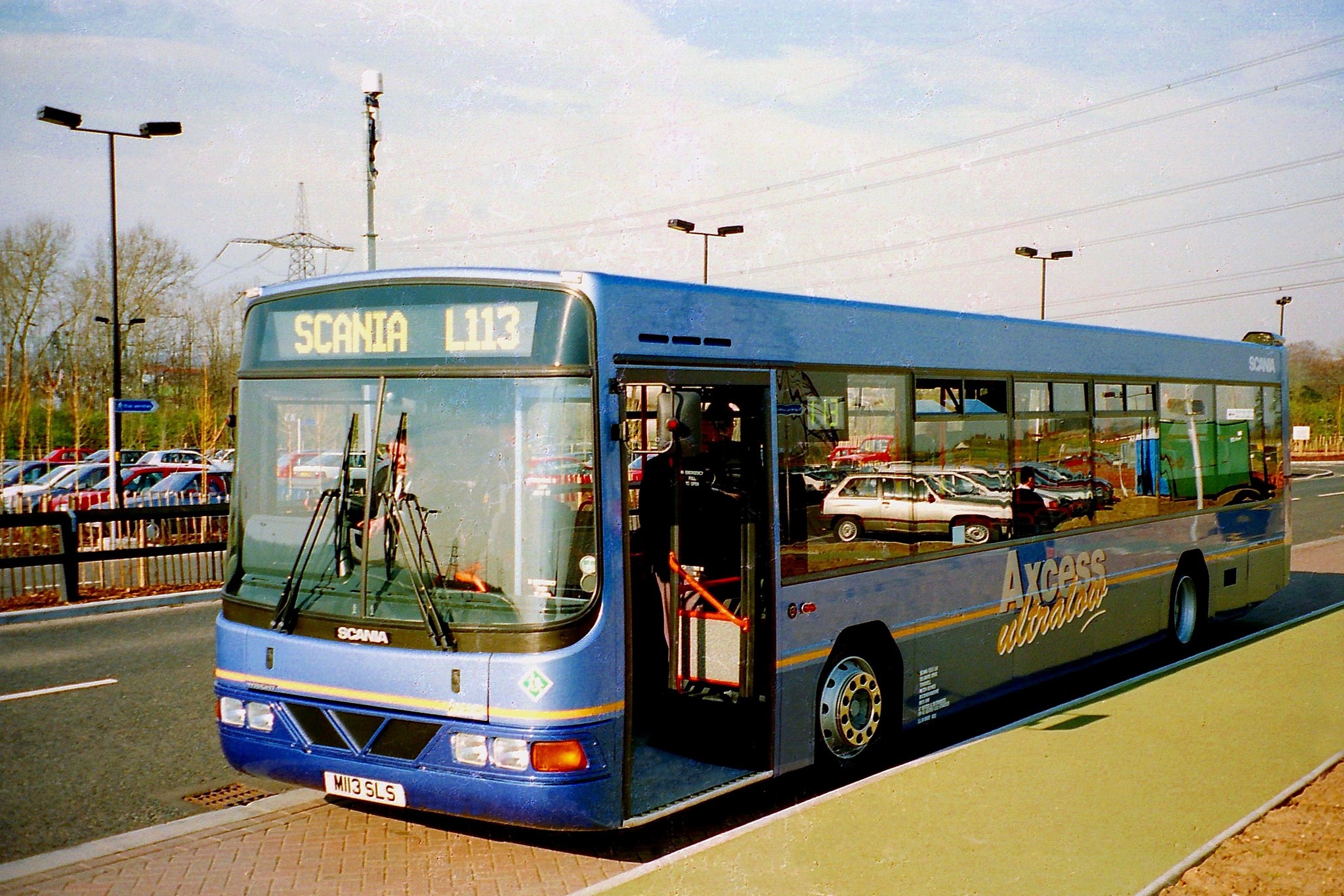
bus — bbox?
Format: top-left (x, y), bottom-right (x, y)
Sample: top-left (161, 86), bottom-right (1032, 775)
top-left (214, 265), bottom-right (1296, 833)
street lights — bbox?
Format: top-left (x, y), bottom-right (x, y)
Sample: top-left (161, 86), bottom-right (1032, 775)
top-left (1016, 246), bottom-right (1072, 320)
top-left (34, 106), bottom-right (184, 536)
top-left (360, 69), bottom-right (385, 274)
top-left (1277, 297), bottom-right (1293, 339)
top-left (668, 219), bottom-right (743, 286)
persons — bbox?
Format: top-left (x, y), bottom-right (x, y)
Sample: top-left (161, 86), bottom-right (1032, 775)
top-left (1010, 465), bottom-right (1055, 536)
top-left (639, 404), bottom-right (741, 599)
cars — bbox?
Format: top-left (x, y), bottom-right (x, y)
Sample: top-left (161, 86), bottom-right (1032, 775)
top-left (271, 449), bottom-right (661, 512)
top-left (0, 446), bottom-right (237, 541)
top-left (758, 443), bottom-right (813, 467)
top-left (827, 434), bottom-right (895, 465)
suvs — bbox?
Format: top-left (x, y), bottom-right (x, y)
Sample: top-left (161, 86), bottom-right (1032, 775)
top-left (784, 463), bottom-right (1113, 546)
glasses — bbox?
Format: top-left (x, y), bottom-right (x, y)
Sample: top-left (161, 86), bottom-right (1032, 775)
top-left (729, 420), bottom-right (738, 427)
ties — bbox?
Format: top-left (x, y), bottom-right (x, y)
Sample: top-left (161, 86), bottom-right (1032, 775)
top-left (398, 476), bottom-right (407, 500)
